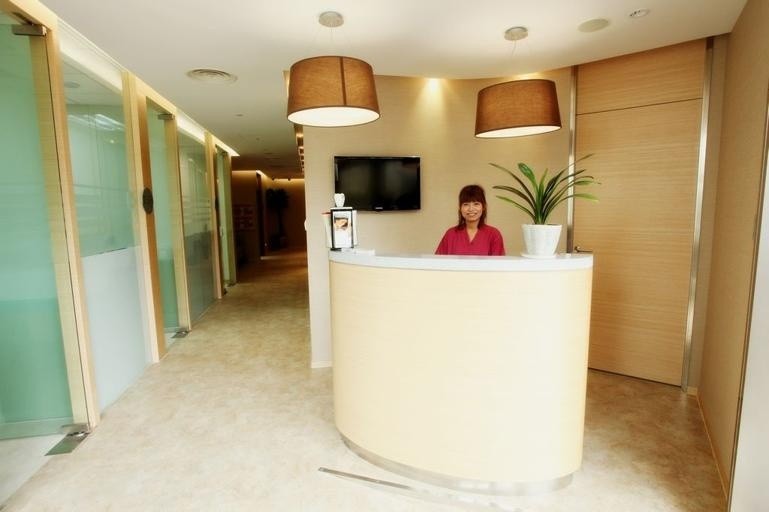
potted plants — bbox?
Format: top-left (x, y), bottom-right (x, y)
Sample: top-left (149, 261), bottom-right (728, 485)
top-left (488, 152), bottom-right (603, 257)
top-left (265, 188), bottom-right (291, 248)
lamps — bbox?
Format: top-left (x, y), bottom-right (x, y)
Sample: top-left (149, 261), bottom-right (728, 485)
top-left (474, 27), bottom-right (561, 139)
top-left (285, 11), bottom-right (380, 129)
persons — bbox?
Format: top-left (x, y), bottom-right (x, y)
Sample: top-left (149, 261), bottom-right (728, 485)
top-left (435, 185), bottom-right (505, 256)
